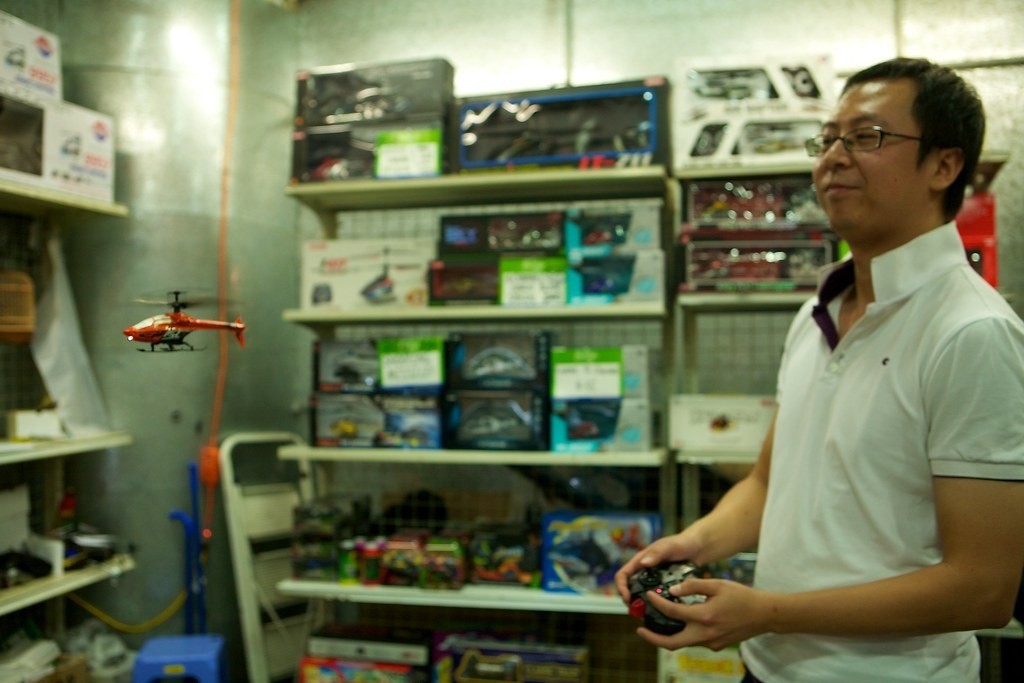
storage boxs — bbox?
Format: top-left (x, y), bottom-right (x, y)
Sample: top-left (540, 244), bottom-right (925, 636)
top-left (283, 57), bottom-right (841, 682)
top-left (0, 10), bottom-right (116, 205)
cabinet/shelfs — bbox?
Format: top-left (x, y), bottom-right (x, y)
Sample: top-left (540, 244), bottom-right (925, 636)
top-left (673, 150), bottom-right (1022, 683)
top-left (283, 165), bottom-right (680, 682)
top-left (1, 178), bottom-right (136, 683)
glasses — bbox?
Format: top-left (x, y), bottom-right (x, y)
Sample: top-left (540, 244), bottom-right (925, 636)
top-left (805, 125), bottom-right (925, 157)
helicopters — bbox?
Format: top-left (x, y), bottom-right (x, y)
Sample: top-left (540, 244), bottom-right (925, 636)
top-left (121, 291), bottom-right (246, 354)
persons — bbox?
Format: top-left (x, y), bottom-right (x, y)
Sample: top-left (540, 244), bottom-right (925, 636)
top-left (615, 56), bottom-right (1024, 683)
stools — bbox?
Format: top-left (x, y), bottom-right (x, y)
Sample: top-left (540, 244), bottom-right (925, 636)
top-left (132, 635), bottom-right (224, 683)
